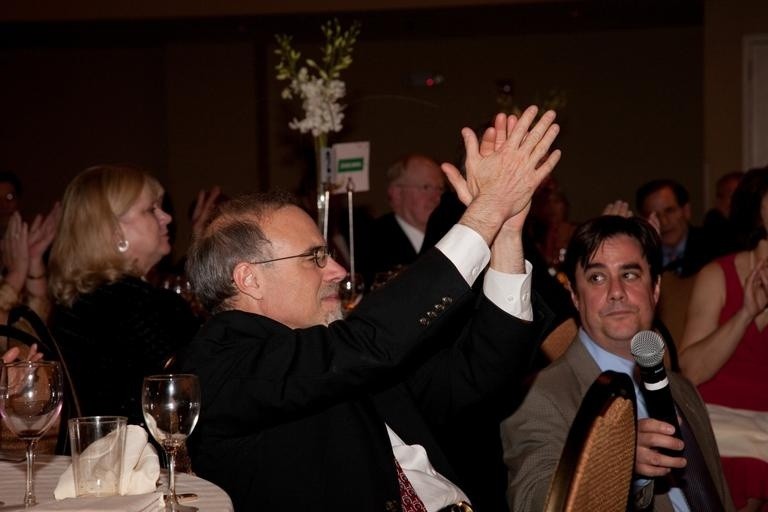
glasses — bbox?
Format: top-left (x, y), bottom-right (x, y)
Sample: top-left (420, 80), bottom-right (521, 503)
top-left (251, 247), bottom-right (336, 268)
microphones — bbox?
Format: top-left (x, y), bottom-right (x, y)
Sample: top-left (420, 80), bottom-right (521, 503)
top-left (630, 331), bottom-right (686, 460)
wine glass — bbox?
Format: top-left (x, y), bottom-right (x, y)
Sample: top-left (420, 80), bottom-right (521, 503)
top-left (0, 358), bottom-right (66, 511)
top-left (141, 372), bottom-right (203, 512)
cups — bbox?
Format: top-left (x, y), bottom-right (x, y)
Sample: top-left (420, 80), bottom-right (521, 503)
top-left (67, 415), bottom-right (130, 498)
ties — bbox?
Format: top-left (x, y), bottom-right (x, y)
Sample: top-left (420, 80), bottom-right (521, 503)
top-left (394, 455), bottom-right (427, 512)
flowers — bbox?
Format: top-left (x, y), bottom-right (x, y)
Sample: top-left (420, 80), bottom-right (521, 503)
top-left (273, 19), bottom-right (363, 192)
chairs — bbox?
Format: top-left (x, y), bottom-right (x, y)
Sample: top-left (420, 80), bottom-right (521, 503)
top-left (542, 371), bottom-right (637, 512)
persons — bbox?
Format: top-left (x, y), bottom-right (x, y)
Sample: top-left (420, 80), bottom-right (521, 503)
top-left (176, 103), bottom-right (562, 512)
top-left (0, 156), bottom-right (768, 512)
